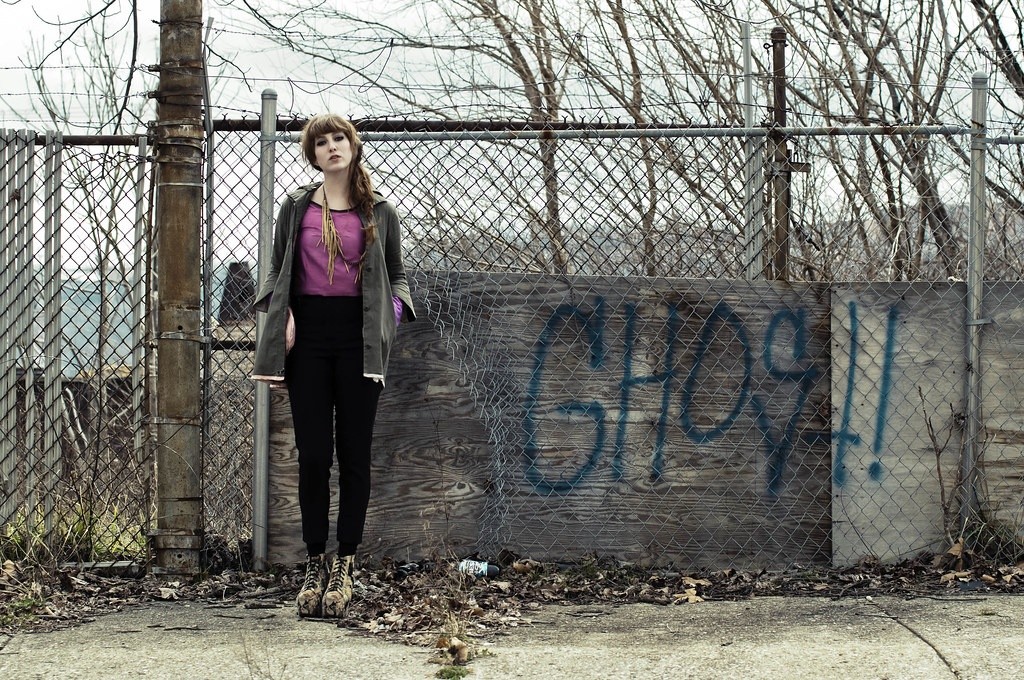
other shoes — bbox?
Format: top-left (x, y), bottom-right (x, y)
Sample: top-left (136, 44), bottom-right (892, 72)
top-left (296, 554), bottom-right (355, 618)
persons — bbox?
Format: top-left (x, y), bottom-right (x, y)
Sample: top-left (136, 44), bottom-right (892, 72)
top-left (264, 113), bottom-right (409, 616)
